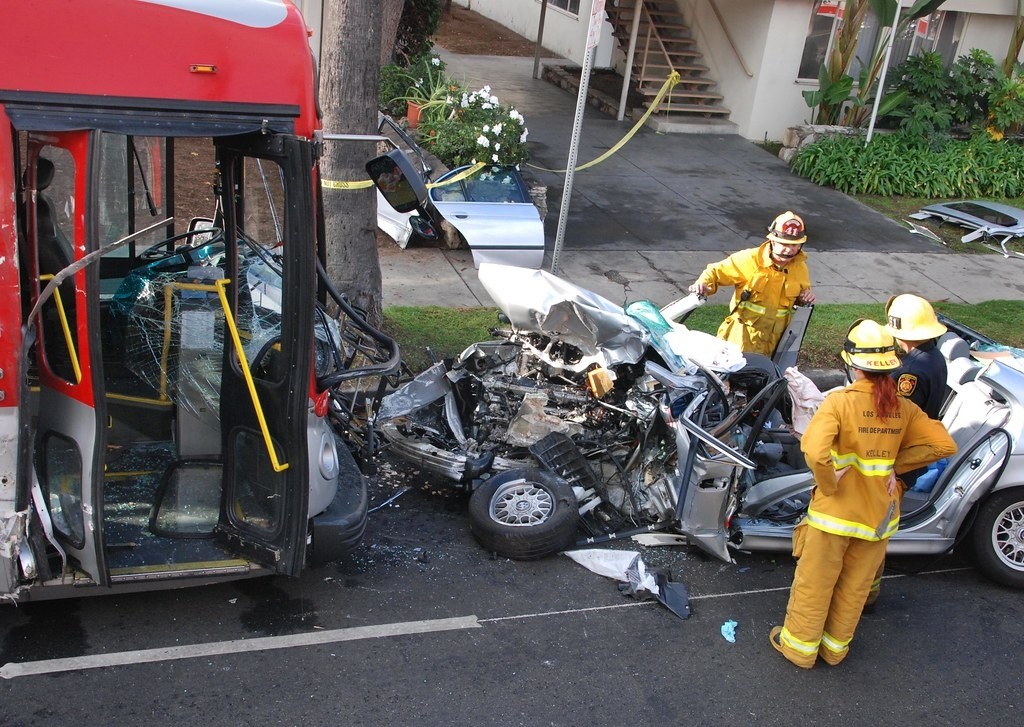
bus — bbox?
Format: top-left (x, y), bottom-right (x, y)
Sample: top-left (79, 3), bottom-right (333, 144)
top-left (1, 1), bottom-right (432, 610)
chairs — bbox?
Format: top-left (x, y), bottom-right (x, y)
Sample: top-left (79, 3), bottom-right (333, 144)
top-left (22, 159), bottom-right (135, 320)
top-left (901, 328), bottom-right (1010, 511)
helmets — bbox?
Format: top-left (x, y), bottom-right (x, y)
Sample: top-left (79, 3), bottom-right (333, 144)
top-left (840, 319), bottom-right (902, 373)
top-left (766, 211), bottom-right (807, 244)
top-left (884, 294), bottom-right (948, 341)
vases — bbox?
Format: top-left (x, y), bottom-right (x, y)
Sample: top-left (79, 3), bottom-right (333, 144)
top-left (429, 129), bottom-right (438, 146)
top-left (406, 101), bottom-right (424, 128)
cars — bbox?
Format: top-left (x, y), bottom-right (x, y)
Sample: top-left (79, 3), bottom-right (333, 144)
top-left (374, 256), bottom-right (1023, 594)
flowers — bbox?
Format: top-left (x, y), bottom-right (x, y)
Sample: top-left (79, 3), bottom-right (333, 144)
top-left (405, 51), bottom-right (529, 184)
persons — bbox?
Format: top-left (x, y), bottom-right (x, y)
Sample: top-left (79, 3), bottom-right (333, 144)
top-left (769, 294), bottom-right (958, 668)
top-left (688, 211), bottom-right (817, 359)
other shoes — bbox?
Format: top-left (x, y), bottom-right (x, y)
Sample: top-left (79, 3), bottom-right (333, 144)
top-left (770, 627), bottom-right (786, 651)
